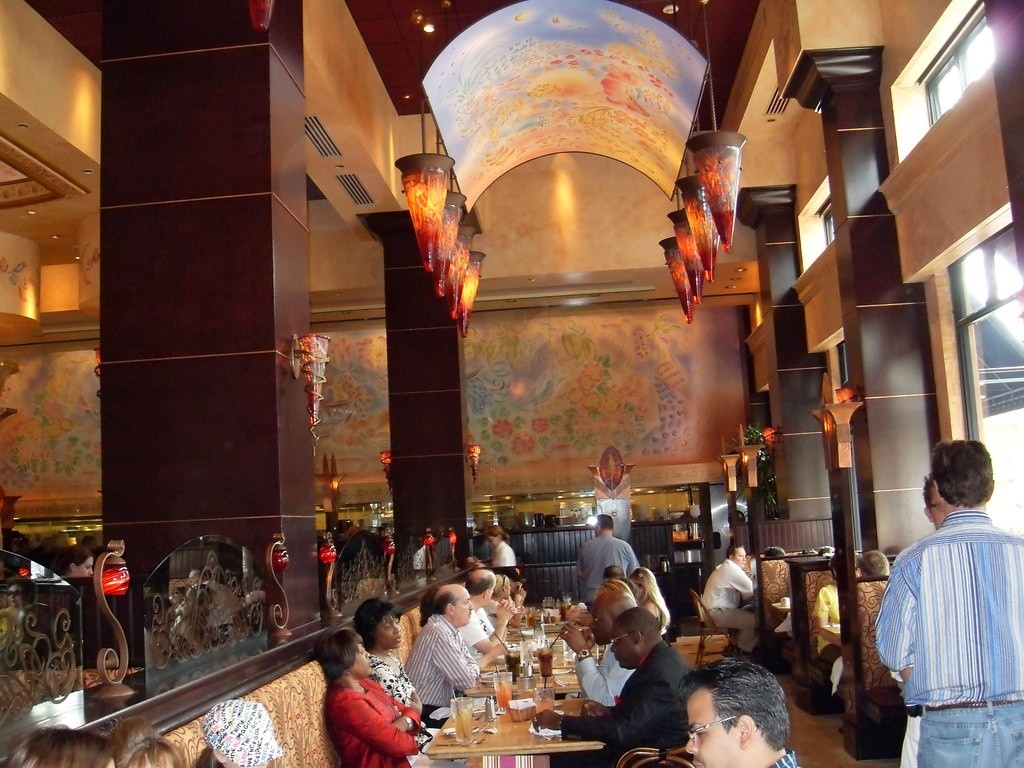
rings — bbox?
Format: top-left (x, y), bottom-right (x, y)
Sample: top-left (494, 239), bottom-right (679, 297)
top-left (533, 716), bottom-right (537, 722)
top-left (564, 630), bottom-right (568, 635)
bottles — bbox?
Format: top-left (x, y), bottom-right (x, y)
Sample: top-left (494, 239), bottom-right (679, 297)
top-left (484, 695), bottom-right (499, 721)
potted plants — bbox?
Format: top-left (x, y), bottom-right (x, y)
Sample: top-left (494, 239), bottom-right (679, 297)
top-left (721, 422), bottom-right (779, 520)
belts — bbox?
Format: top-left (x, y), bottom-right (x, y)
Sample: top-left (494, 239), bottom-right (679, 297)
top-left (925, 700), bottom-right (1015, 711)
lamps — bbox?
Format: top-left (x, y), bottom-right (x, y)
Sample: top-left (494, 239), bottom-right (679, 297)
top-left (423, 528), bottom-right (437, 582)
top-left (394, 28), bottom-right (455, 271)
top-left (674, 147), bottom-right (722, 283)
top-left (835, 385), bottom-right (863, 430)
top-left (382, 527), bottom-right (400, 595)
top-left (464, 443), bottom-right (481, 485)
top-left (266, 533), bottom-right (293, 638)
top-left (320, 531), bottom-right (343, 618)
top-left (445, 527), bottom-right (461, 573)
top-left (762, 425), bottom-right (783, 458)
top-left (667, 189), bottom-right (704, 305)
top-left (659, 228), bottom-right (695, 324)
top-left (457, 251), bottom-right (485, 337)
top-left (93, 539), bottom-right (135, 698)
top-left (685, 0), bottom-right (748, 253)
top-left (288, 332), bottom-right (330, 456)
top-left (433, 130), bottom-right (467, 299)
top-left (380, 451), bottom-right (393, 497)
top-left (448, 223), bottom-right (476, 321)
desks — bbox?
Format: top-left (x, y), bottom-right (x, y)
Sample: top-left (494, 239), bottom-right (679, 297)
top-left (426, 609), bottom-right (607, 768)
top-left (771, 603), bottom-right (792, 613)
top-left (821, 625), bottom-right (842, 648)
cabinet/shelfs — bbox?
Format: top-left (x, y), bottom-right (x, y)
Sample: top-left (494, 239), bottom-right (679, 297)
top-left (671, 517), bottom-right (704, 566)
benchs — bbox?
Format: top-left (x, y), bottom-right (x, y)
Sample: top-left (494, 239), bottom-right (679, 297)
top-left (154, 578), bottom-right (467, 768)
top-left (756, 551), bottom-right (908, 761)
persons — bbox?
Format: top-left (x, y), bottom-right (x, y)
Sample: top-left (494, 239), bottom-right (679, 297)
top-left (890, 469), bottom-right (946, 768)
top-left (0, 699), bottom-right (285, 768)
top-left (316, 514), bottom-right (697, 768)
top-left (0, 531), bottom-right (96, 646)
top-left (700, 543), bottom-right (862, 663)
top-left (837, 549), bottom-right (890, 735)
top-left (675, 658), bottom-right (805, 768)
top-left (142, 565), bottom-right (266, 668)
top-left (873, 439), bottom-right (1024, 768)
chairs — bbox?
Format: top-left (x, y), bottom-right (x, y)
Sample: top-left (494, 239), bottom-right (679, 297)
top-left (616, 743), bottom-right (694, 768)
top-left (690, 589), bottom-right (740, 666)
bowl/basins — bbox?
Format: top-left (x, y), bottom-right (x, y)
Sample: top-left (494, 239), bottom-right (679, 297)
top-left (482, 672), bottom-right (497, 681)
top-left (480, 681), bottom-right (495, 688)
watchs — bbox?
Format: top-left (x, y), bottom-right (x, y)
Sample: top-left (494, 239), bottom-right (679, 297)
top-left (578, 649), bottom-right (592, 659)
top-left (402, 716), bottom-right (413, 728)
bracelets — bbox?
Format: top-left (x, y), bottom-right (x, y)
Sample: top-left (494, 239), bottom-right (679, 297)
top-left (577, 617), bottom-right (580, 624)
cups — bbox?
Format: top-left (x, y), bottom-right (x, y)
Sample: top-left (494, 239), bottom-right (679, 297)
top-left (450, 697), bottom-right (474, 745)
top-left (493, 672), bottom-right (513, 707)
top-left (780, 596), bottom-right (790, 607)
top-left (525, 607), bottom-right (535, 629)
top-left (542, 591), bottom-right (572, 621)
top-left (533, 687), bottom-right (555, 714)
top-left (563, 638), bottom-right (577, 662)
top-left (504, 651), bottom-right (520, 685)
top-left (537, 648), bottom-right (554, 678)
top-left (518, 511), bottom-right (556, 528)
top-left (520, 640), bottom-right (533, 665)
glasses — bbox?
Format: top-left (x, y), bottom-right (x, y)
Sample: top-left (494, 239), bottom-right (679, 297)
top-left (687, 716), bottom-right (736, 745)
top-left (929, 502), bottom-right (944, 512)
top-left (611, 631), bottom-right (644, 647)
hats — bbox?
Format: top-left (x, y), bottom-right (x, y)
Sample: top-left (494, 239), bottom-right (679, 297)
top-left (203, 700), bottom-right (285, 767)
top-left (506, 567), bottom-right (526, 581)
